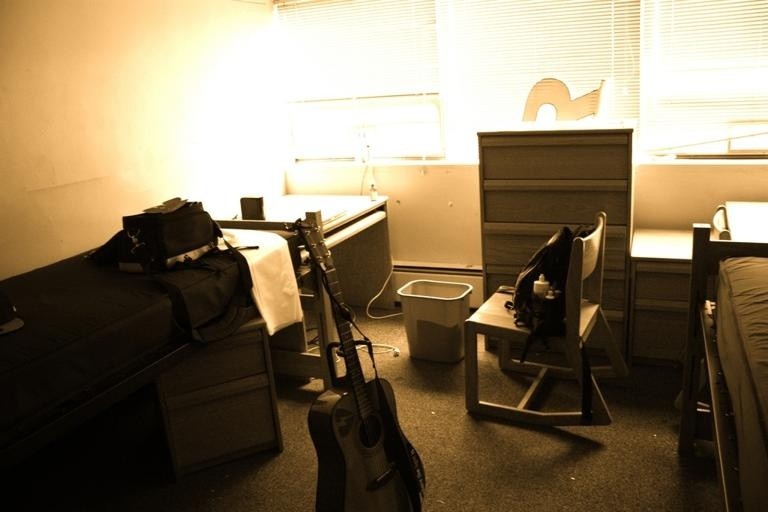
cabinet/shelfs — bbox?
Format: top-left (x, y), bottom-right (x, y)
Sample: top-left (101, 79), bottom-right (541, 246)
top-left (632, 228), bottom-right (694, 366)
top-left (477, 130), bottom-right (633, 364)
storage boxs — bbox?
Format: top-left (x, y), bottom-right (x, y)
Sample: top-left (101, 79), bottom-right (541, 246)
top-left (129, 318), bottom-right (282, 481)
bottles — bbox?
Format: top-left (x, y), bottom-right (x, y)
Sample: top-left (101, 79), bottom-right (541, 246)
top-left (533, 272), bottom-right (551, 332)
top-left (546, 290), bottom-right (554, 299)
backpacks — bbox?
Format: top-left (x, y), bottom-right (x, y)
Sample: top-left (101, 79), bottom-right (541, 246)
top-left (504, 224), bottom-right (595, 334)
top-left (120, 198), bottom-right (253, 344)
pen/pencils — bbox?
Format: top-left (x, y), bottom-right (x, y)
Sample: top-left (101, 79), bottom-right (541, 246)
top-left (238, 246), bottom-right (259, 250)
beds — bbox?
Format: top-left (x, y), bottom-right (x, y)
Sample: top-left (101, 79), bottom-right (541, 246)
top-left (680, 225), bottom-right (768, 512)
top-left (0, 209), bottom-right (338, 512)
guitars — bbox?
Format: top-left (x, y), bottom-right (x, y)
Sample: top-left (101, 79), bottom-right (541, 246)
top-left (294, 218), bottom-right (425, 511)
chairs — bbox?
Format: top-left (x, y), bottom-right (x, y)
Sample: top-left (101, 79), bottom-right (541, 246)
top-left (675, 206), bottom-right (731, 416)
top-left (463, 211), bottom-right (630, 428)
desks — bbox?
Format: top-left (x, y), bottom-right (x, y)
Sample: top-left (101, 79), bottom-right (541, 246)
top-left (230, 195), bottom-right (394, 390)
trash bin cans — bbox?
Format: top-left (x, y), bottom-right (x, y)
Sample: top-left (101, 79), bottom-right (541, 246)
top-left (396, 280), bottom-right (474, 363)
